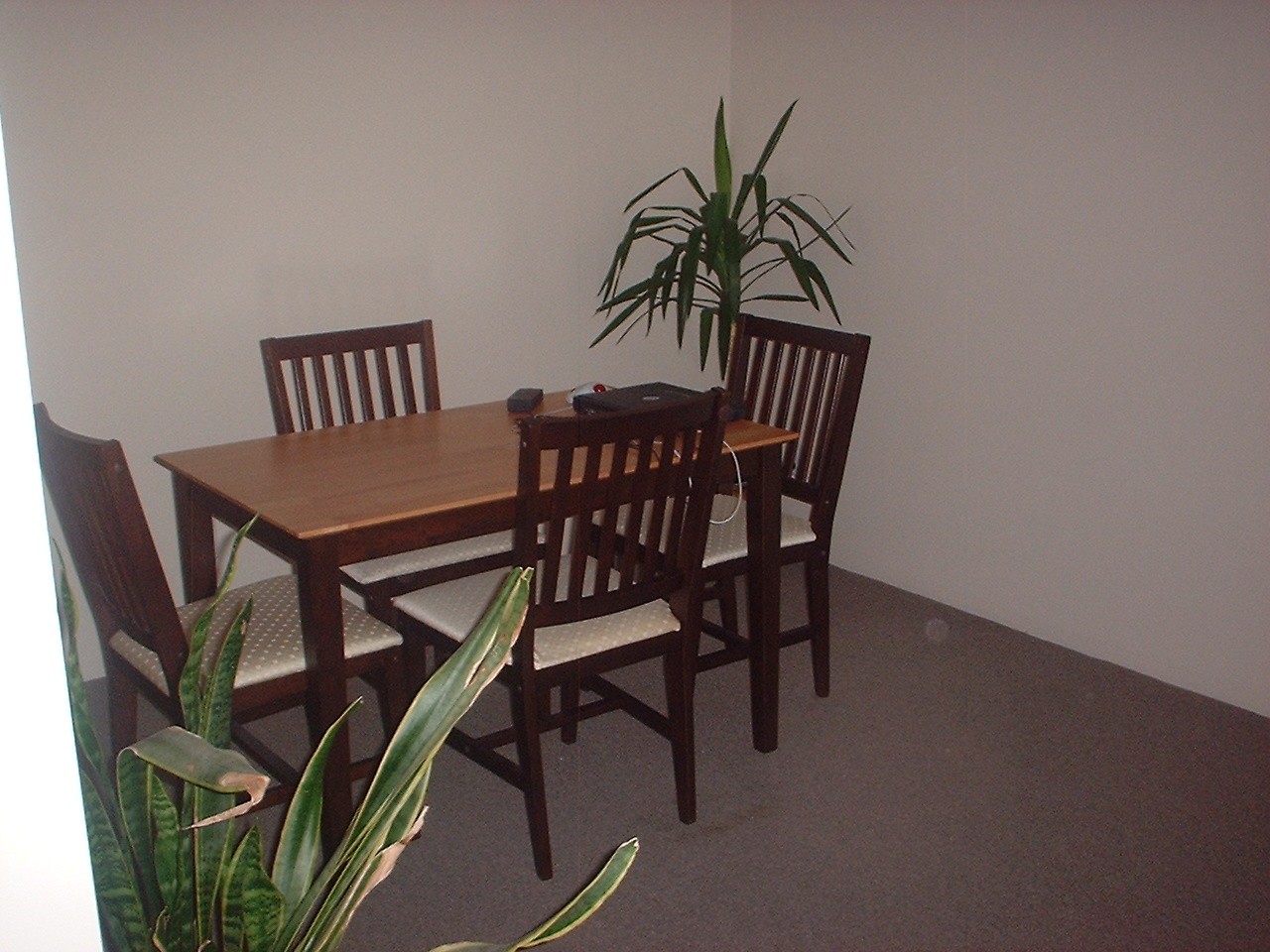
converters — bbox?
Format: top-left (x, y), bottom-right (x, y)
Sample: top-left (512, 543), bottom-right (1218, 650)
top-left (507, 388), bottom-right (543, 413)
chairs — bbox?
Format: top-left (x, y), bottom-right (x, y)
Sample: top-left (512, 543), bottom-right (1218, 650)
top-left (260, 319), bottom-right (546, 626)
top-left (34, 403), bottom-right (413, 861)
top-left (588, 312), bottom-right (871, 696)
top-left (390, 385), bottom-right (732, 881)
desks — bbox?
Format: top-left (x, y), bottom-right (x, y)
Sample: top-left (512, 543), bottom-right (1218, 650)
top-left (152, 390), bottom-right (799, 888)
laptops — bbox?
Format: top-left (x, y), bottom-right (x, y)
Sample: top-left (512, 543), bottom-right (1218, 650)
top-left (572, 382), bottom-right (704, 415)
